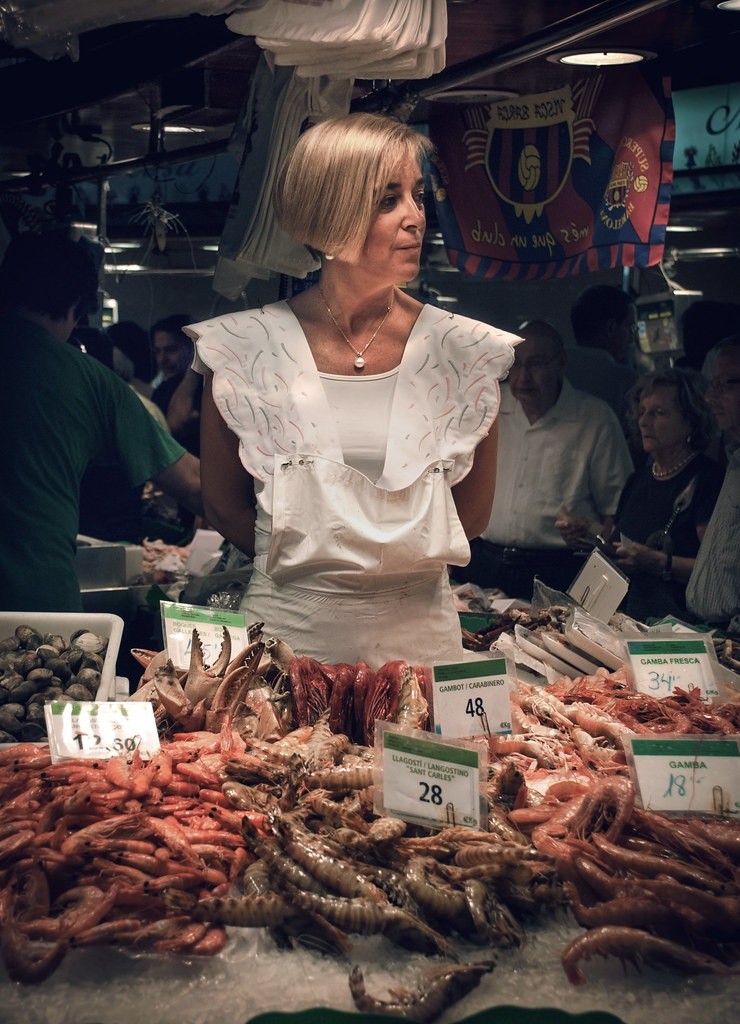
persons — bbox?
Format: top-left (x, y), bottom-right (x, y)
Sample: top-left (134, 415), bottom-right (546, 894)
top-left (179, 111), bottom-right (526, 671)
top-left (1, 230), bottom-right (740, 634)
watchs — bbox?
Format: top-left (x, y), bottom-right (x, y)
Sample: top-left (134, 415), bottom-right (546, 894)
top-left (663, 554), bottom-right (674, 582)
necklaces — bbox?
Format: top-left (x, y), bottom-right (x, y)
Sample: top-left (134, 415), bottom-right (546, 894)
top-left (319, 286), bottom-right (396, 370)
top-left (648, 453), bottom-right (698, 477)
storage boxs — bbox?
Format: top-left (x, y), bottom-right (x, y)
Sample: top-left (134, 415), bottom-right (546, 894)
top-left (0, 611), bottom-right (124, 750)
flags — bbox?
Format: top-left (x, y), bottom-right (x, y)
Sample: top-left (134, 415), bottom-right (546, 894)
top-left (428, 50), bottom-right (676, 280)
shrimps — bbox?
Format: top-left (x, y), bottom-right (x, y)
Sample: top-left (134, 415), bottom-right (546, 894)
top-left (2, 614), bottom-right (740, 1024)
top-left (346, 964), bottom-right (494, 1024)
